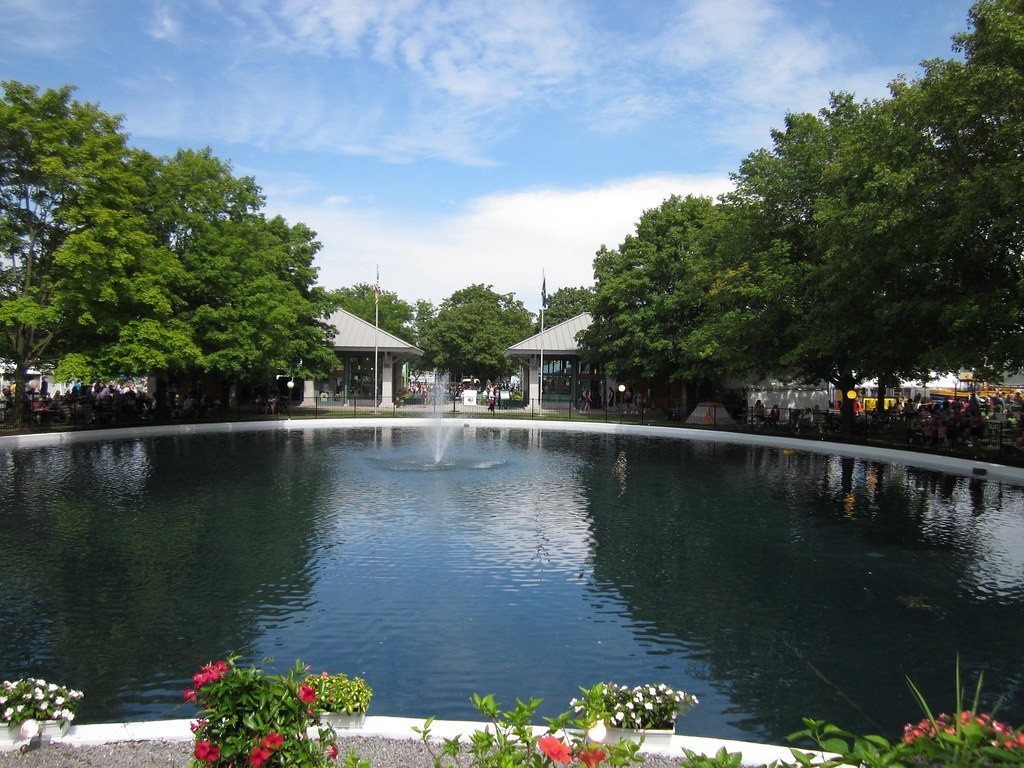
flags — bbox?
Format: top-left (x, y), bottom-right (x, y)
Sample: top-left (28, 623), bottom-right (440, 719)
top-left (374, 276), bottom-right (380, 302)
top-left (540, 278), bottom-right (546, 308)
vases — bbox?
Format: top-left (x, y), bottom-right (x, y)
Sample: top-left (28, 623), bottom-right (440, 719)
top-left (0, 719), bottom-right (70, 738)
top-left (606, 723), bottom-right (677, 743)
top-left (317, 704), bottom-right (369, 728)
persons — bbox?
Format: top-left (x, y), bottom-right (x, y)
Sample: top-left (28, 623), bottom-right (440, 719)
top-left (168, 384), bottom-right (212, 417)
top-left (891, 390), bottom-right (1022, 447)
top-left (50, 380), bottom-right (156, 414)
top-left (496, 381), bottom-right (515, 392)
top-left (255, 394), bottom-right (276, 414)
top-left (857, 400), bottom-right (862, 412)
top-left (861, 388), bottom-right (866, 397)
top-left (803, 408), bottom-right (813, 423)
top-left (41, 377), bottom-right (47, 393)
top-left (6, 386), bottom-right (11, 399)
top-left (409, 381), bottom-right (480, 396)
top-left (608, 387), bottom-right (618, 412)
top-left (579, 387), bottom-right (592, 413)
top-left (742, 395), bottom-right (748, 424)
top-left (813, 405), bottom-right (820, 415)
top-left (754, 400), bottom-right (780, 428)
top-left (623, 389), bottom-right (643, 414)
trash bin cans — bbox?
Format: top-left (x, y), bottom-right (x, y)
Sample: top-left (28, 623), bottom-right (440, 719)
top-left (943, 441), bottom-right (978, 459)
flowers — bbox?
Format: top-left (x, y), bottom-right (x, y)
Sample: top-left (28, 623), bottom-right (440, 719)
top-left (902, 711), bottom-right (1024, 751)
top-left (302, 673), bottom-right (373, 715)
top-left (171, 646), bottom-right (373, 768)
top-left (0, 678), bottom-right (84, 738)
top-left (411, 692), bottom-right (648, 768)
top-left (569, 681), bottom-right (699, 728)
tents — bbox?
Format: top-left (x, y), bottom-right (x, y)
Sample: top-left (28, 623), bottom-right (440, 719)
top-left (687, 403), bottom-right (736, 424)
